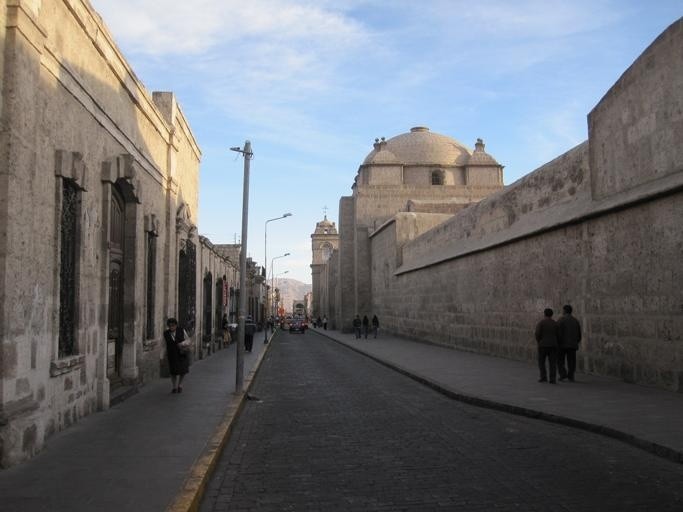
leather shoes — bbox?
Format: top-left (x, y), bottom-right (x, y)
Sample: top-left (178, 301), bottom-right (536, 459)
top-left (538, 376), bottom-right (575, 384)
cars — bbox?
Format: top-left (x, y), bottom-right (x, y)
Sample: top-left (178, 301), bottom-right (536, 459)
top-left (270, 315), bottom-right (309, 335)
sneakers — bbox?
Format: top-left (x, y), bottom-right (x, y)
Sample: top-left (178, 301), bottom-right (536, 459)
top-left (172, 388), bottom-right (177, 393)
top-left (177, 387), bottom-right (182, 393)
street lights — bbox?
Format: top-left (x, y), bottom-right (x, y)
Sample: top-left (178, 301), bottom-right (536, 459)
top-left (274, 270), bottom-right (290, 328)
top-left (264, 212), bottom-right (293, 344)
top-left (271, 253), bottom-right (291, 333)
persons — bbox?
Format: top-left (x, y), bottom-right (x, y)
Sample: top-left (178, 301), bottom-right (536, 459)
top-left (222, 313), bottom-right (230, 344)
top-left (163, 318), bottom-right (189, 393)
top-left (535, 308), bottom-right (559, 384)
top-left (363, 315), bottom-right (369, 339)
top-left (372, 315), bottom-right (379, 339)
top-left (557, 305), bottom-right (581, 383)
top-left (245, 315), bottom-right (254, 353)
top-left (353, 315), bottom-right (361, 339)
top-left (312, 313), bottom-right (329, 330)
